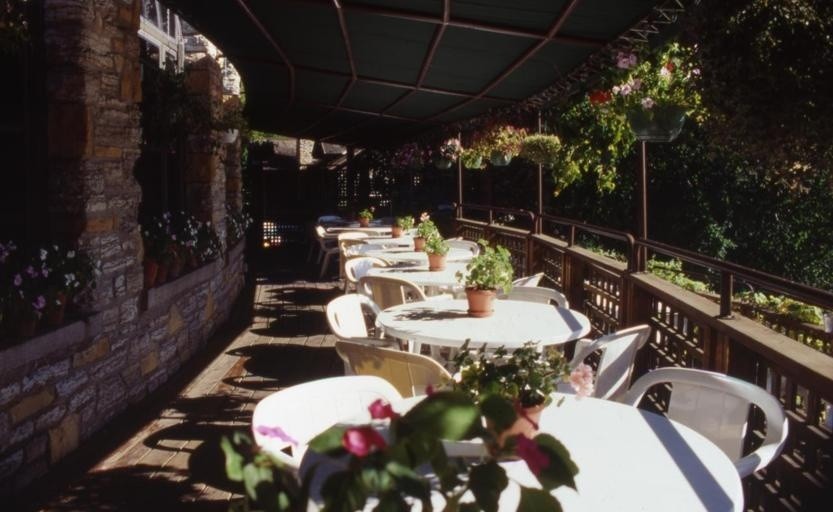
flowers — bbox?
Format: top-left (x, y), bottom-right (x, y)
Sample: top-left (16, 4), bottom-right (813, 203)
top-left (135, 209), bottom-right (225, 276)
top-left (1, 238), bottom-right (97, 324)
top-left (219, 329), bottom-right (596, 509)
top-left (395, 38), bottom-right (709, 200)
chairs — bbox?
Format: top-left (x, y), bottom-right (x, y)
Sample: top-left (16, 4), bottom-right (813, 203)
top-left (251, 215), bottom-right (788, 482)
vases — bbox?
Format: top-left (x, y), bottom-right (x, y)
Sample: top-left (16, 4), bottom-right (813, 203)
top-left (141, 255), bottom-right (180, 289)
top-left (2, 308), bottom-right (37, 341)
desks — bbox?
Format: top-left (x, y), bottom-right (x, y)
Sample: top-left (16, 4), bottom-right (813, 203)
top-left (307, 387), bottom-right (748, 511)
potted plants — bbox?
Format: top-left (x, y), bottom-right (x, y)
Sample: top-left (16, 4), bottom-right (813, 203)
top-left (357, 209), bottom-right (374, 228)
